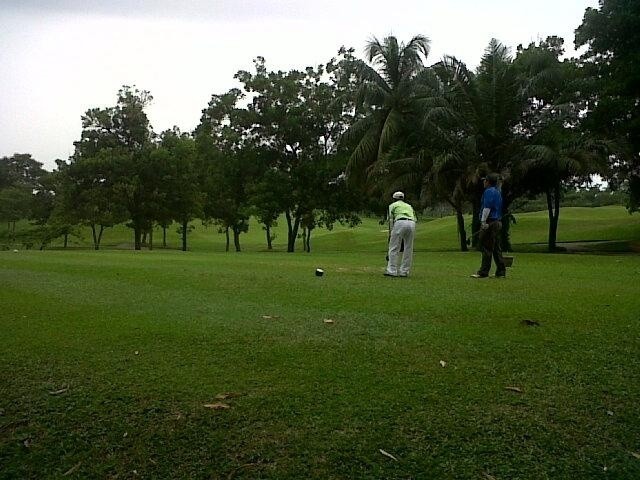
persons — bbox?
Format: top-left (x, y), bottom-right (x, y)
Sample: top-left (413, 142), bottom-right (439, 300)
top-left (384, 191), bottom-right (417, 276)
top-left (468, 174), bottom-right (507, 278)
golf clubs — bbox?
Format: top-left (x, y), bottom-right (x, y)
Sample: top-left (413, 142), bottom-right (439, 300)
top-left (466, 202), bottom-right (530, 245)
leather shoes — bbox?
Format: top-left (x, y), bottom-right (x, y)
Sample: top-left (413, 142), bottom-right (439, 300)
top-left (470, 274), bottom-right (487, 278)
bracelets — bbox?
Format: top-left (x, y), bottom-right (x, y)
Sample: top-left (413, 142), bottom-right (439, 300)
top-left (480, 220), bottom-right (487, 223)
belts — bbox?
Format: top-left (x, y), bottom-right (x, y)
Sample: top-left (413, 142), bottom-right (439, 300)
top-left (397, 217), bottom-right (412, 220)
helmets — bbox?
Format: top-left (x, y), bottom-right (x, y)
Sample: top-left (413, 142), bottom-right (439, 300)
top-left (393, 192), bottom-right (404, 200)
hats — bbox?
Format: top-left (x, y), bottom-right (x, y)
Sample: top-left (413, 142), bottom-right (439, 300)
top-left (480, 173), bottom-right (498, 186)
